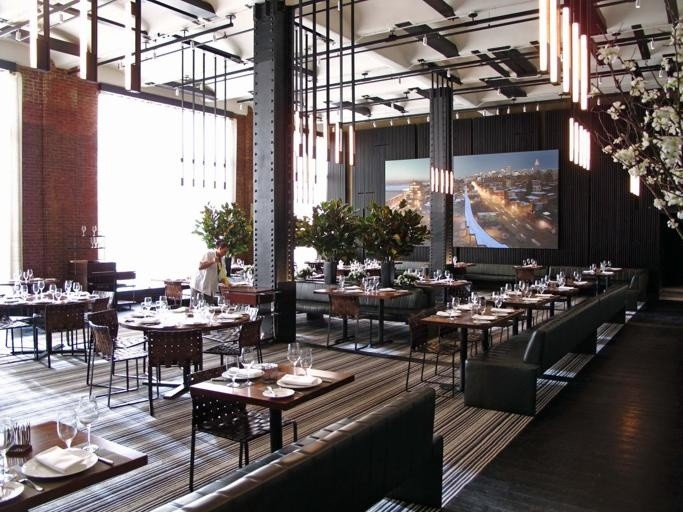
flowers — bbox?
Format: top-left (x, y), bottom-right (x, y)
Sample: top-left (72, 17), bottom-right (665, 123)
top-left (584, 10), bottom-right (683, 248)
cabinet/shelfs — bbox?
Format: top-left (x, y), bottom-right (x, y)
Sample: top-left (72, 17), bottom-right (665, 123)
top-left (72, 260), bottom-right (116, 292)
top-left (225, 286), bottom-right (281, 342)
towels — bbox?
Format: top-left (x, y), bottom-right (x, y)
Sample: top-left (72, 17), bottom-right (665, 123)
top-left (472, 313), bottom-right (496, 321)
top-left (522, 297), bottom-right (542, 301)
top-left (281, 373), bottom-right (317, 385)
top-left (132, 310), bottom-right (156, 317)
top-left (217, 313), bottom-right (242, 319)
top-left (35, 445), bottom-right (84, 474)
top-left (35, 301), bottom-right (51, 304)
top-left (534, 294), bottom-right (554, 297)
top-left (4, 300), bottom-right (18, 303)
top-left (490, 307), bottom-right (515, 313)
top-left (229, 367), bottom-right (261, 376)
top-left (173, 306), bottom-right (188, 312)
top-left (139, 317), bottom-right (160, 326)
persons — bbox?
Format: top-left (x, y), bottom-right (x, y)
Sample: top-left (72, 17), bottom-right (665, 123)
top-left (190, 241), bottom-right (229, 308)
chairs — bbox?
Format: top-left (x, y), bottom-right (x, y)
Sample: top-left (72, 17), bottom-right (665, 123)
top-left (484, 299), bottom-right (513, 346)
top-left (144, 330), bottom-right (203, 415)
top-left (84, 309), bottom-right (147, 390)
top-left (406, 309), bottom-right (461, 398)
top-left (162, 280), bottom-right (183, 304)
top-left (186, 363), bottom-right (296, 493)
top-left (433, 303), bottom-right (474, 375)
top-left (90, 289), bottom-right (114, 309)
top-left (326, 293), bottom-right (368, 351)
top-left (87, 320), bottom-right (159, 409)
top-left (513, 303), bottom-right (535, 331)
top-left (202, 305), bottom-right (260, 342)
top-left (204, 318), bottom-right (264, 366)
top-left (0, 294), bottom-right (32, 355)
top-left (24, 301), bottom-right (86, 368)
top-left (66, 296), bottom-right (110, 346)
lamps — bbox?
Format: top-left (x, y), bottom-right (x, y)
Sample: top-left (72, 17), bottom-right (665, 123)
top-left (566, 98), bottom-right (594, 172)
top-left (16, 30), bottom-right (22, 41)
top-left (153, 49), bottom-right (156, 59)
top-left (498, 88), bottom-right (500, 95)
top-left (597, 97), bottom-right (600, 106)
top-left (240, 103), bottom-right (242, 110)
top-left (496, 107), bottom-right (499, 115)
top-left (523, 104), bottom-right (527, 113)
top-left (200, 82), bottom-right (203, 91)
top-left (427, 114), bottom-right (430, 121)
top-left (58, 13), bottom-right (63, 23)
top-left (190, 41), bottom-right (194, 49)
top-left (390, 120), bottom-right (393, 126)
top-left (659, 69), bottom-right (663, 77)
top-left (212, 35), bottom-right (216, 42)
top-left (407, 117), bottom-right (410, 124)
top-left (447, 67), bottom-right (450, 78)
top-left (507, 106), bottom-right (510, 114)
top-left (373, 121), bottom-right (376, 128)
top-left (423, 35), bottom-right (427, 46)
top-left (418, 58), bottom-right (462, 195)
top-left (176, 87), bottom-right (180, 96)
top-left (456, 112), bottom-right (459, 120)
top-left (293, 0), bottom-right (363, 167)
top-left (536, 103), bottom-right (539, 111)
top-left (483, 111), bottom-right (486, 116)
top-left (535, 0), bottom-right (595, 112)
top-left (666, 93), bottom-right (669, 98)
top-left (636, 0), bottom-right (640, 7)
top-left (651, 38), bottom-right (655, 49)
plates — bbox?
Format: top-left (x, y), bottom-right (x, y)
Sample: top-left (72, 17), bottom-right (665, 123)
top-left (275, 376), bottom-right (323, 389)
top-left (0, 480), bottom-right (25, 504)
top-left (263, 387), bottom-right (295, 400)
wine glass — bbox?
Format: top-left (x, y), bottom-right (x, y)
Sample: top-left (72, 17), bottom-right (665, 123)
top-left (491, 257), bottom-right (612, 310)
top-left (237, 258), bottom-right (253, 283)
top-left (13, 268), bottom-right (81, 304)
top-left (225, 342), bottom-right (313, 389)
top-left (339, 274), bottom-right (346, 290)
top-left (362, 275), bottom-right (380, 296)
top-left (402, 267), bottom-right (454, 284)
top-left (140, 293), bottom-right (231, 325)
top-left (446, 291), bottom-right (487, 323)
top-left (337, 258), bottom-right (381, 270)
top-left (0, 394), bottom-right (99, 481)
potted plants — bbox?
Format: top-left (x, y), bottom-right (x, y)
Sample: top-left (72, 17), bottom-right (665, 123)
top-left (191, 201), bottom-right (252, 277)
top-left (294, 198), bottom-right (368, 285)
top-left (363, 198), bottom-right (433, 287)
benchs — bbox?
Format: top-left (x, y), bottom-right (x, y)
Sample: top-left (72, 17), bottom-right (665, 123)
top-left (547, 266), bottom-right (649, 312)
top-left (294, 283), bottom-right (428, 353)
top-left (464, 285), bottom-right (628, 416)
top-left (466, 264), bottom-right (547, 290)
top-left (148, 385), bottom-right (444, 511)
top-left (393, 261), bottom-right (430, 276)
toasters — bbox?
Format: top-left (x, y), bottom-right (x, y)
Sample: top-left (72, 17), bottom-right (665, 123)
top-left (80, 224), bottom-right (99, 249)
